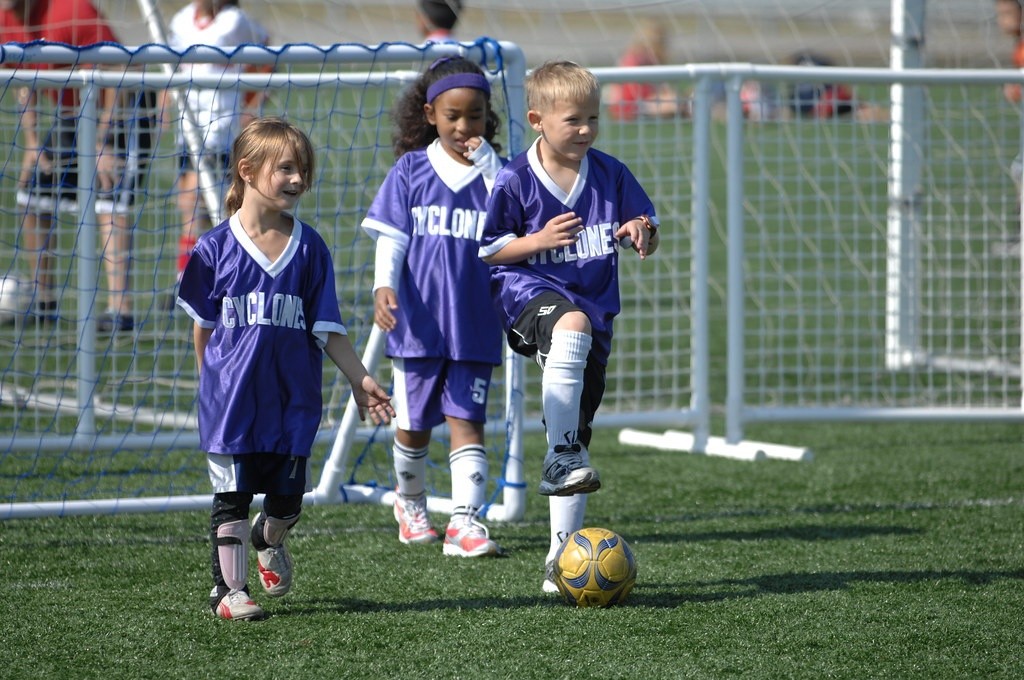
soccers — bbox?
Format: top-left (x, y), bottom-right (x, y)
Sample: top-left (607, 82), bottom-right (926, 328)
top-left (555, 528), bottom-right (638, 610)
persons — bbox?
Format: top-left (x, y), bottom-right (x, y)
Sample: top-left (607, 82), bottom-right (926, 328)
top-left (994, 2), bottom-right (1022, 258)
top-left (477, 61), bottom-right (661, 594)
top-left (359, 56), bottom-right (510, 557)
top-left (1, 0), bottom-right (157, 334)
top-left (157, 2), bottom-right (270, 311)
top-left (177, 117), bottom-right (396, 620)
top-left (607, 17), bottom-right (682, 121)
top-left (416, 2), bottom-right (465, 41)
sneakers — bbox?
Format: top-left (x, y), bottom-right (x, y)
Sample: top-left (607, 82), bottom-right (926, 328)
top-left (542, 559), bottom-right (561, 595)
top-left (250, 511), bottom-right (293, 597)
top-left (209, 583), bottom-right (264, 622)
top-left (97, 309), bottom-right (141, 334)
top-left (442, 519), bottom-right (503, 558)
top-left (538, 443), bottom-right (602, 497)
top-left (392, 485), bottom-right (439, 544)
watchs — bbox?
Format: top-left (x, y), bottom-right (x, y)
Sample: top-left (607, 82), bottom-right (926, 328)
top-left (633, 215), bottom-right (657, 246)
